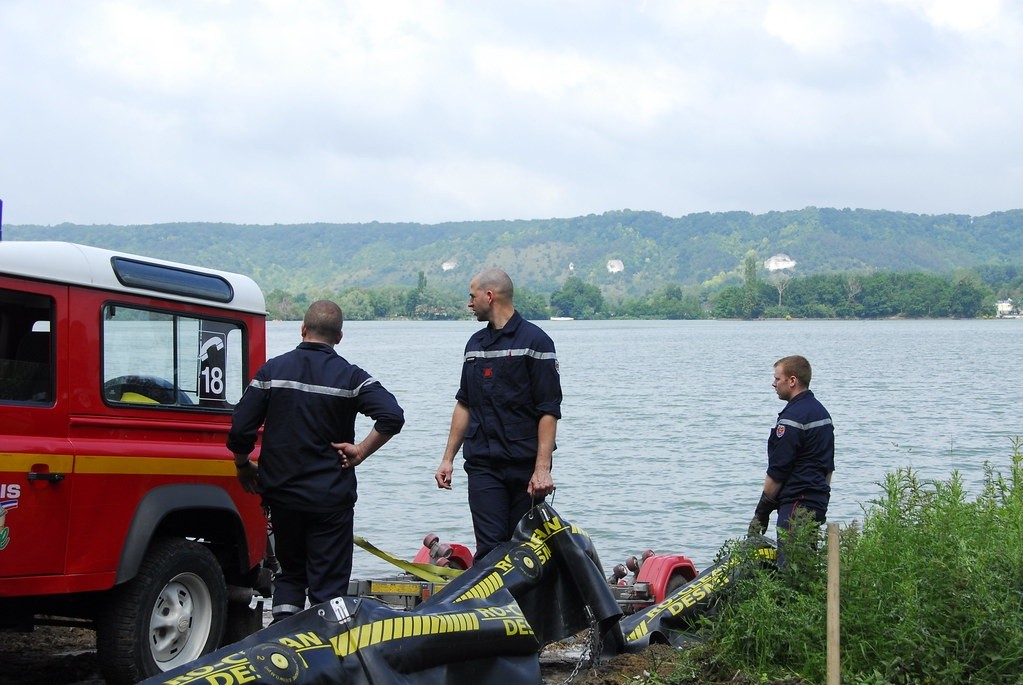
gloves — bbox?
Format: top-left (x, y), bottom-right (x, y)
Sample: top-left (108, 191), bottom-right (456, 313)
top-left (235, 460), bottom-right (260, 495)
top-left (747, 491), bottom-right (778, 538)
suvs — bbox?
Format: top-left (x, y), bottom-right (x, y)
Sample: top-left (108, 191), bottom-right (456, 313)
top-left (0, 240), bottom-right (268, 685)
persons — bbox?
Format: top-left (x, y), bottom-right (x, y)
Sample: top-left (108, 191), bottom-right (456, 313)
top-left (225, 300), bottom-right (404, 622)
top-left (747, 355), bottom-right (836, 593)
top-left (435, 268), bottom-right (562, 565)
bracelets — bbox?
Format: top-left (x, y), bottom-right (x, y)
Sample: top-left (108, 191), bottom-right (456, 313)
top-left (235, 459), bottom-right (250, 468)
